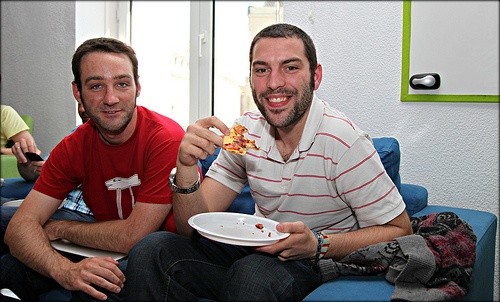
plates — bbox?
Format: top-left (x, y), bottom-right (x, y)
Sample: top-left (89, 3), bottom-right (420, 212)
top-left (1, 200), bottom-right (69, 210)
top-left (186, 211), bottom-right (291, 247)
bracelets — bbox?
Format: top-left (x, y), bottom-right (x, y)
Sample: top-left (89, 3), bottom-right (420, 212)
top-left (312, 230), bottom-right (331, 264)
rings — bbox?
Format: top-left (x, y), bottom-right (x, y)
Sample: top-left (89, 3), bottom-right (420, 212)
top-left (14, 147), bottom-right (20, 150)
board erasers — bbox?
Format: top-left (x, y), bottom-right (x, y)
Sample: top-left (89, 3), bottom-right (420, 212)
top-left (410, 72), bottom-right (441, 90)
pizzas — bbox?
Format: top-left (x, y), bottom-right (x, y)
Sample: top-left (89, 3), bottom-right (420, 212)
top-left (222, 125), bottom-right (260, 155)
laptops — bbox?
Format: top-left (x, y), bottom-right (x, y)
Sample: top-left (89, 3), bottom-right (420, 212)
top-left (50, 238), bottom-right (128, 262)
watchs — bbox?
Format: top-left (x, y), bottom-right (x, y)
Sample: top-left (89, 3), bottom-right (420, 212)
top-left (168, 168), bottom-right (201, 194)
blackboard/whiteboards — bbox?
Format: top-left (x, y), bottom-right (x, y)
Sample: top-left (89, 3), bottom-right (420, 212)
top-left (400, 0), bottom-right (500, 103)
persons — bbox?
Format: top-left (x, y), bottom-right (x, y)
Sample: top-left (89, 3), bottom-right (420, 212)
top-left (1, 102), bottom-right (96, 253)
top-left (1, 38), bottom-right (203, 301)
top-left (122, 24), bottom-right (412, 302)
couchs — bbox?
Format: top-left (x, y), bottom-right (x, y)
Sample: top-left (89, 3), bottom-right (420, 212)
top-left (0, 114), bottom-right (497, 302)
